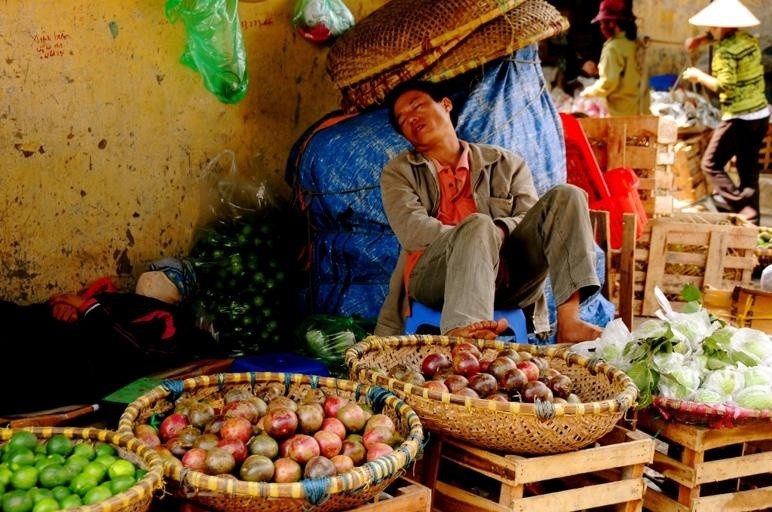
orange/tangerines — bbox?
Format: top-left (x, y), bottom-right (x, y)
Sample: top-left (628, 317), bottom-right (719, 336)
top-left (0, 432), bottom-right (146, 512)
top-left (192, 222), bottom-right (284, 343)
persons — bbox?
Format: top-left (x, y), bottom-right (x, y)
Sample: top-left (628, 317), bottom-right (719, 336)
top-left (682, 0), bottom-right (771, 224)
top-left (380, 79), bottom-right (603, 344)
top-left (0, 257), bottom-right (201, 417)
top-left (580, 0), bottom-right (651, 115)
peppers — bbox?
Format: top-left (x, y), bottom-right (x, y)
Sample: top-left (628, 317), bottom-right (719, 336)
top-left (290, 0), bottom-right (332, 43)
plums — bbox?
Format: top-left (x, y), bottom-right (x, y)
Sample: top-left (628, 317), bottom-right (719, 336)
top-left (135, 387), bottom-right (395, 482)
top-left (423, 342), bottom-right (580, 405)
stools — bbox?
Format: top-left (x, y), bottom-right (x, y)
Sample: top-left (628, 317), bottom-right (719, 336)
top-left (400, 299), bottom-right (531, 345)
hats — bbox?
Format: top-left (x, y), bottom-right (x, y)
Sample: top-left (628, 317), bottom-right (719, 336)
top-left (689, 0), bottom-right (761, 28)
top-left (592, 0), bottom-right (626, 24)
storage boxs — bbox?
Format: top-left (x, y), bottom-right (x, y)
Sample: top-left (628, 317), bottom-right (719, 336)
top-left (635, 401), bottom-right (771, 512)
top-left (566, 108), bottom-right (772, 320)
top-left (427, 417), bottom-right (656, 511)
